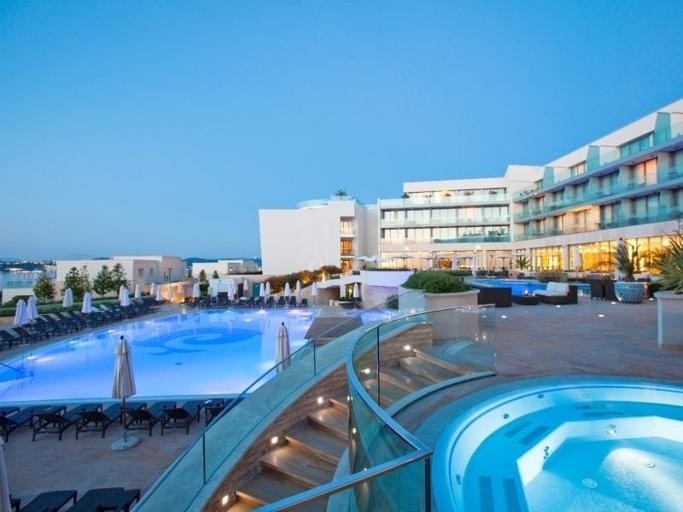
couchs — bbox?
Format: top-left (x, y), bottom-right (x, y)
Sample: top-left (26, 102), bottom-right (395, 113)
top-left (532, 282), bottom-right (577, 305)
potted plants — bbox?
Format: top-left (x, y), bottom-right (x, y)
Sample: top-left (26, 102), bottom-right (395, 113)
top-left (614, 227), bottom-right (683, 350)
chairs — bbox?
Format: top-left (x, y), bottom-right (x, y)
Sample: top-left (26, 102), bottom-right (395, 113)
top-left (0, 398), bottom-right (225, 443)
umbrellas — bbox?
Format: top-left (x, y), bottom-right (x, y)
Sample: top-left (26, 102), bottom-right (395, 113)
top-left (111, 336), bottom-right (136, 441)
top-left (26, 296), bottom-right (39, 320)
top-left (355, 251), bottom-right (530, 278)
top-left (615, 239), bottom-right (629, 280)
top-left (0, 436), bottom-right (13, 511)
top-left (276, 321), bottom-right (290, 376)
top-left (79, 292), bottom-right (91, 317)
top-left (13, 299), bottom-right (30, 343)
top-left (62, 288), bottom-right (73, 313)
top-left (115, 278), bottom-right (318, 317)
top-left (352, 283), bottom-right (359, 308)
top-left (572, 247), bottom-right (584, 277)
top-left (339, 282), bottom-right (346, 299)
